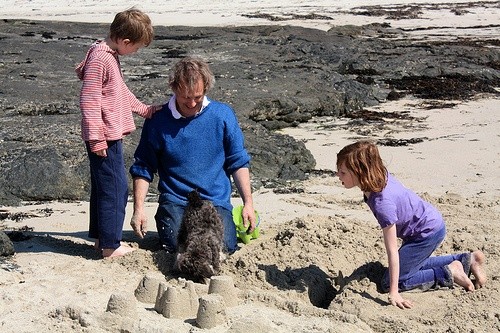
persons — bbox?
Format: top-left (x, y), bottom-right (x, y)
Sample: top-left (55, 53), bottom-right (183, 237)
top-left (73, 7), bottom-right (164, 258)
top-left (336, 140), bottom-right (488, 311)
top-left (129, 57), bottom-right (257, 259)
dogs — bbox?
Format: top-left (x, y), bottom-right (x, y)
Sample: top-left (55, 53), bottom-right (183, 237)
top-left (175, 191), bottom-right (224, 284)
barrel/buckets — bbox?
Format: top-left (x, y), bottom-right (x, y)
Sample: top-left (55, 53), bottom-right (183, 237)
top-left (232, 204), bottom-right (261, 245)
